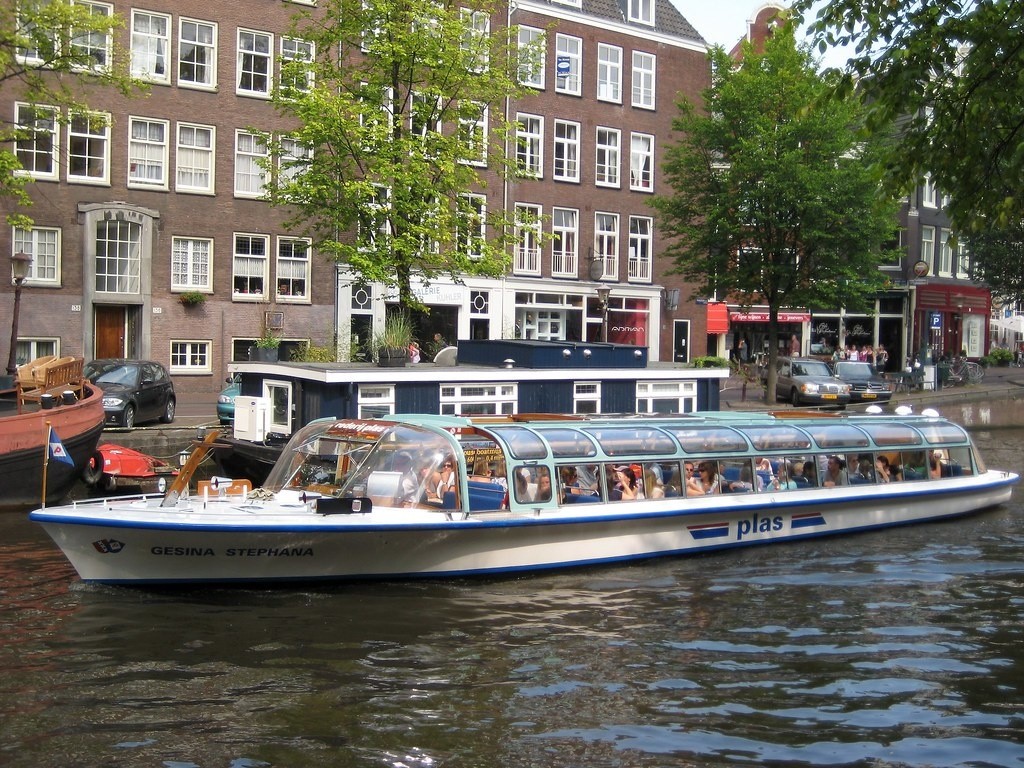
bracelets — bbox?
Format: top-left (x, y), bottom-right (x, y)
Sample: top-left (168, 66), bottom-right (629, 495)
top-left (442, 482), bottom-right (448, 485)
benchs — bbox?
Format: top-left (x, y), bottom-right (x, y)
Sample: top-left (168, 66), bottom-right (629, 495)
top-left (562, 486), bottom-right (601, 503)
top-left (460, 481), bottom-right (504, 511)
top-left (662, 458), bottom-right (962, 497)
top-left (894, 381), bottom-right (934, 395)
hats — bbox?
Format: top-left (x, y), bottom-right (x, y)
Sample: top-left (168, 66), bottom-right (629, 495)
top-left (394, 451), bottom-right (412, 461)
top-left (613, 466), bottom-right (628, 471)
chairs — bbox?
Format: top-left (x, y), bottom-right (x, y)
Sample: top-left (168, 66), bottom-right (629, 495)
top-left (14, 355), bottom-right (84, 415)
top-left (443, 491), bottom-right (455, 509)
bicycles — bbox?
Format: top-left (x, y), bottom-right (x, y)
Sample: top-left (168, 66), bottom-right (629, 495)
top-left (894, 352), bottom-right (922, 394)
top-left (941, 354), bottom-right (985, 389)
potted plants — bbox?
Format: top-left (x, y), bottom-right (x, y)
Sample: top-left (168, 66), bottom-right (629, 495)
top-left (250, 304), bottom-right (281, 362)
top-left (243, 281), bottom-right (248, 293)
top-left (180, 291), bottom-right (206, 306)
top-left (990, 349), bottom-right (1013, 366)
top-left (374, 306), bottom-right (417, 367)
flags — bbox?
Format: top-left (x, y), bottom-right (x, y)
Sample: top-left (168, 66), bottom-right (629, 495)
top-left (48, 425), bottom-right (74, 466)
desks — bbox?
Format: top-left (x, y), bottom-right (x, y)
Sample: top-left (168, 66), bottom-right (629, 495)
top-left (892, 372), bottom-right (926, 394)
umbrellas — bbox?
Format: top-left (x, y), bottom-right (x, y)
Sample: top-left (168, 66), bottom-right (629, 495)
top-left (990, 315), bottom-right (1024, 333)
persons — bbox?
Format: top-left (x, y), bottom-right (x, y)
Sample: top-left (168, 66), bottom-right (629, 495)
top-left (990, 337), bottom-right (1010, 351)
top-left (394, 334), bottom-right (941, 508)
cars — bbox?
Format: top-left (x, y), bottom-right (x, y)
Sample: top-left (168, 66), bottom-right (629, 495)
top-left (827, 359), bottom-right (893, 406)
top-left (82, 359), bottom-right (176, 427)
top-left (761, 356), bottom-right (851, 411)
top-left (216, 371), bottom-right (243, 425)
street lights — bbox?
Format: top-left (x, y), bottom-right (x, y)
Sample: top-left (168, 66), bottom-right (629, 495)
top-left (595, 282), bottom-right (613, 344)
top-left (4, 249), bottom-right (34, 376)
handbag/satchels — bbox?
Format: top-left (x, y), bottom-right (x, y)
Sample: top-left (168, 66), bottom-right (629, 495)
top-left (877, 353), bottom-right (883, 361)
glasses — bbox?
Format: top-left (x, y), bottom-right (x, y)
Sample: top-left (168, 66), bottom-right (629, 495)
top-left (574, 474), bottom-right (577, 479)
top-left (698, 468), bottom-right (707, 472)
top-left (685, 468), bottom-right (693, 472)
top-left (441, 463), bottom-right (452, 468)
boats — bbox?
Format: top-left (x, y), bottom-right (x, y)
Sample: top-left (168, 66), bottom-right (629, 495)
top-left (197, 338), bottom-right (731, 490)
top-left (29, 405), bottom-right (1020, 585)
top-left (0, 353), bottom-right (107, 511)
top-left (90, 443), bottom-right (181, 497)
